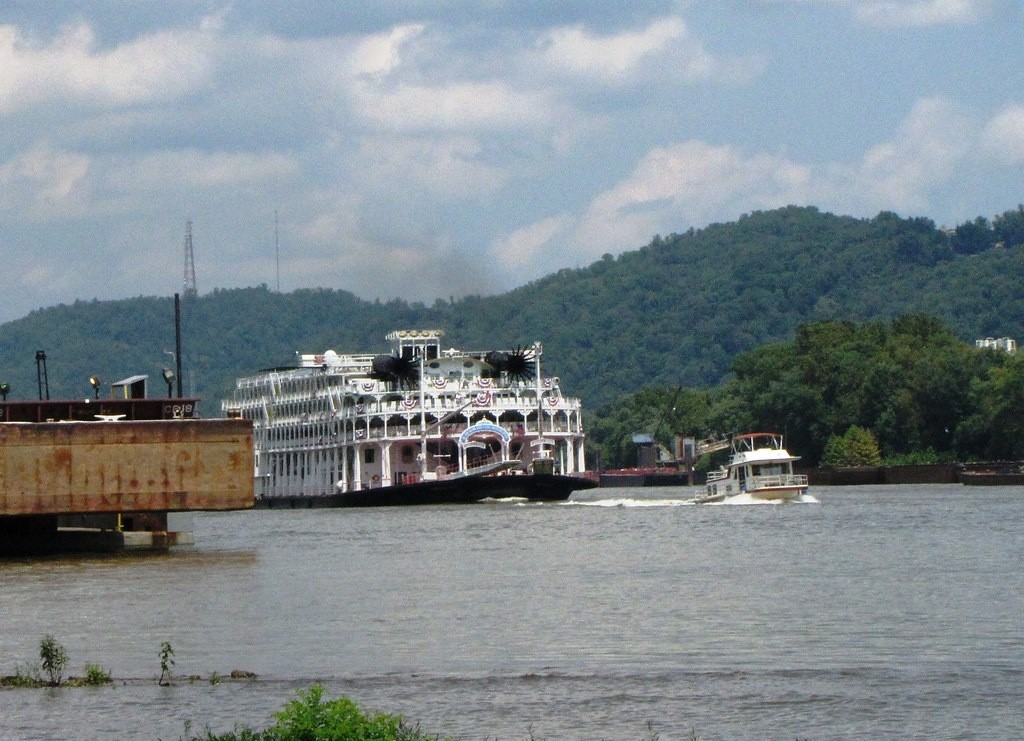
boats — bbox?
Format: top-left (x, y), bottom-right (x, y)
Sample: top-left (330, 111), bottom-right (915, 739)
top-left (689, 431), bottom-right (810, 503)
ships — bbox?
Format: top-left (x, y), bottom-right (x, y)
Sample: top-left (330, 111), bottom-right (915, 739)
top-left (215, 326), bottom-right (600, 503)
